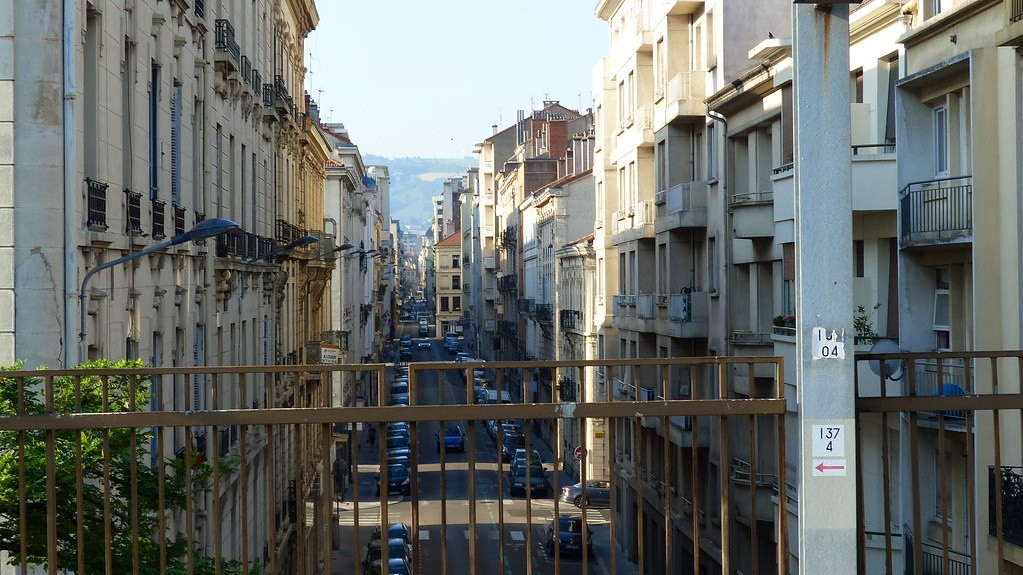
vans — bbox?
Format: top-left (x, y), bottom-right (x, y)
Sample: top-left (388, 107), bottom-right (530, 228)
top-left (443, 332), bottom-right (459, 343)
top-left (485, 390), bottom-right (511, 405)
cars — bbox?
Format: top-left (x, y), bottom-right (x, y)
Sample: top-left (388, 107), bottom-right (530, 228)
top-left (388, 347), bottom-right (413, 472)
top-left (374, 465), bottom-right (411, 497)
top-left (364, 523), bottom-right (413, 575)
top-left (456, 353), bottom-right (485, 382)
top-left (418, 317), bottom-right (429, 337)
top-left (487, 420), bottom-right (533, 462)
top-left (510, 462), bottom-right (547, 497)
top-left (546, 516), bottom-right (593, 556)
top-left (435, 425), bottom-right (466, 453)
top-left (399, 336), bottom-right (412, 347)
top-left (445, 340), bottom-right (462, 353)
top-left (474, 378), bottom-right (488, 404)
top-left (561, 479), bottom-right (609, 510)
top-left (510, 448), bottom-right (545, 475)
top-left (417, 340), bottom-right (432, 350)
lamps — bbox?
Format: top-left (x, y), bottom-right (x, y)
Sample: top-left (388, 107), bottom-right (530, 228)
top-left (308, 244), bottom-right (355, 263)
top-left (80, 219), bottom-right (239, 342)
top-left (334, 249), bottom-right (366, 260)
top-left (354, 249), bottom-right (377, 258)
top-left (367, 253), bottom-right (382, 259)
top-left (247, 236), bottom-right (319, 267)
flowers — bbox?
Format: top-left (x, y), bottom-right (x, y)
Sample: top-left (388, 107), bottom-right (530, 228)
top-left (773, 314), bottom-right (795, 327)
